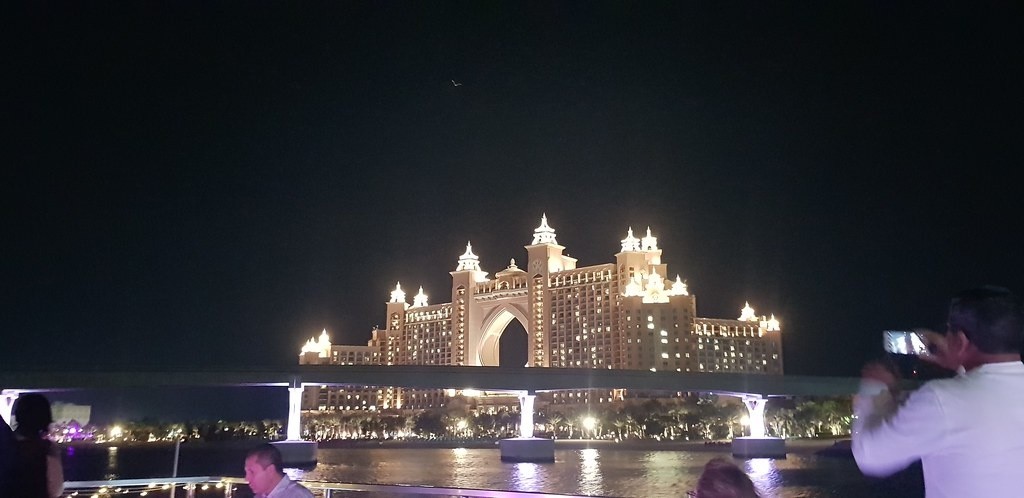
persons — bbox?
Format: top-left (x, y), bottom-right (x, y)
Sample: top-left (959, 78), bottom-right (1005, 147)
top-left (0, 393), bottom-right (64, 498)
top-left (695, 462), bottom-right (758, 498)
top-left (849, 284), bottom-right (1024, 498)
top-left (244, 443), bottom-right (313, 498)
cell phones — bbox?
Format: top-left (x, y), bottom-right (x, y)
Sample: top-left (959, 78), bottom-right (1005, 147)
top-left (883, 330), bottom-right (929, 357)
